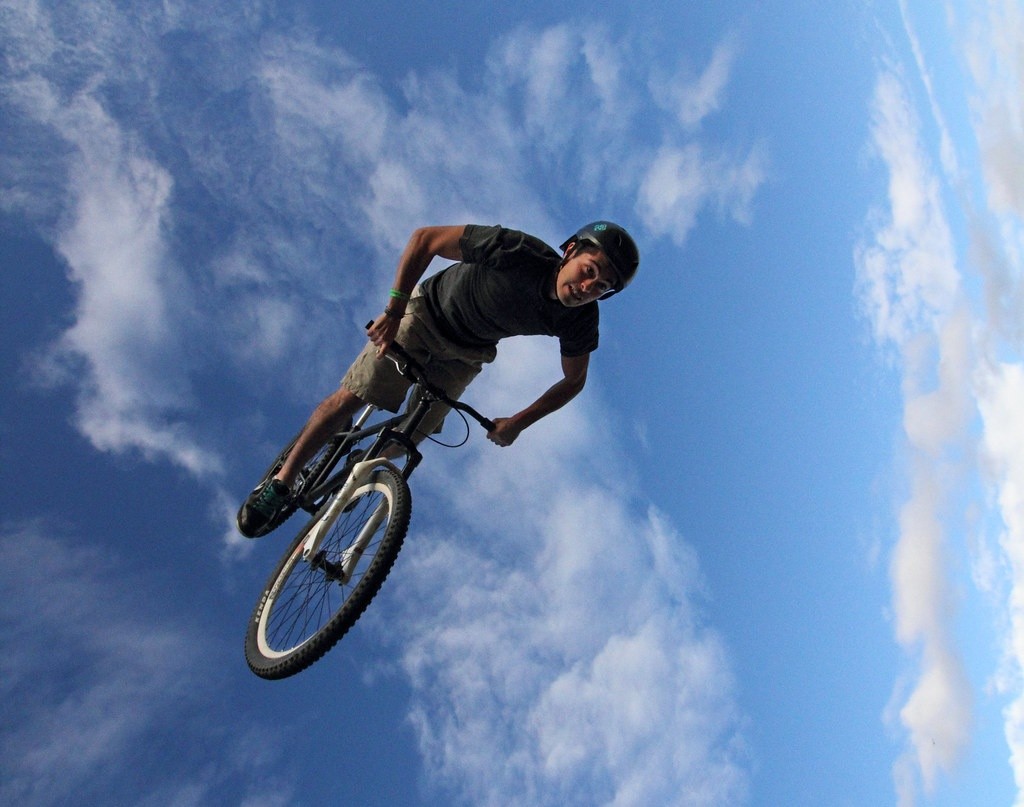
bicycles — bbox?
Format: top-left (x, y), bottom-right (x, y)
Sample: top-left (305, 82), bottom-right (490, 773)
top-left (244, 319), bottom-right (499, 679)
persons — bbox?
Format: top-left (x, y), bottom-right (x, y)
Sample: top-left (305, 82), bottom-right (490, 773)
top-left (236, 221), bottom-right (639, 537)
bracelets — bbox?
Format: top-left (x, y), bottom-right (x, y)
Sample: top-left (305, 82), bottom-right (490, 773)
top-left (390, 289), bottom-right (410, 300)
top-left (384, 306), bottom-right (406, 320)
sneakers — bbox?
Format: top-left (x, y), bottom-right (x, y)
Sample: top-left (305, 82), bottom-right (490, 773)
top-left (337, 450), bottom-right (365, 514)
top-left (236, 478), bottom-right (291, 539)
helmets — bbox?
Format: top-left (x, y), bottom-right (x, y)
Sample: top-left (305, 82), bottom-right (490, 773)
top-left (559, 221), bottom-right (639, 301)
